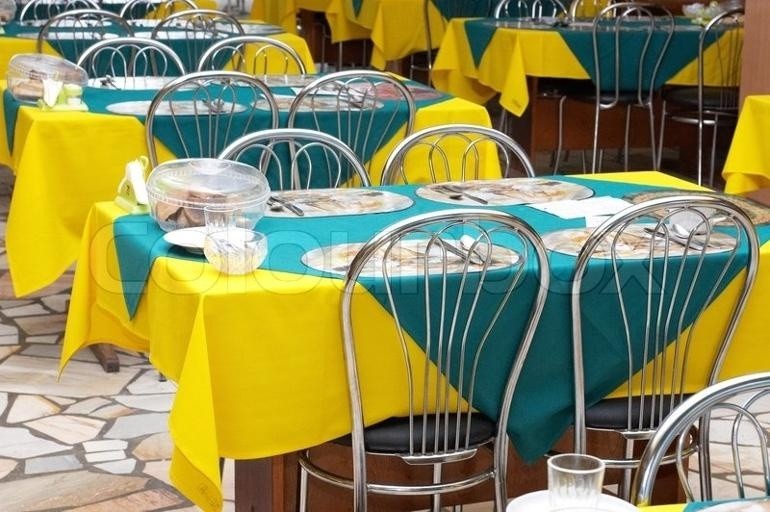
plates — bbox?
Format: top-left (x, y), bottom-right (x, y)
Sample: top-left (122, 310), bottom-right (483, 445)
top-left (505, 489), bottom-right (640, 512)
top-left (162, 226), bottom-right (255, 255)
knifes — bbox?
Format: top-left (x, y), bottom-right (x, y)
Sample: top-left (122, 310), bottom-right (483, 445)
top-left (434, 239), bottom-right (483, 265)
top-left (443, 184), bottom-right (488, 205)
top-left (644, 227), bottom-right (704, 251)
top-left (272, 195), bottom-right (305, 217)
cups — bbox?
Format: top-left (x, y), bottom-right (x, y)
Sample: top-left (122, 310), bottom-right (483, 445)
top-left (547, 452), bottom-right (606, 493)
top-left (203, 205), bottom-right (238, 231)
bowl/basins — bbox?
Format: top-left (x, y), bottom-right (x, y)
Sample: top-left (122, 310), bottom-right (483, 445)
top-left (203, 229), bottom-right (269, 275)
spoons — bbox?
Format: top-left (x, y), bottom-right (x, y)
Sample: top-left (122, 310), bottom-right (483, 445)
top-left (672, 223), bottom-right (721, 249)
top-left (460, 235), bottom-right (487, 263)
top-left (267, 200), bottom-right (282, 211)
top-left (431, 186), bottom-right (462, 198)
